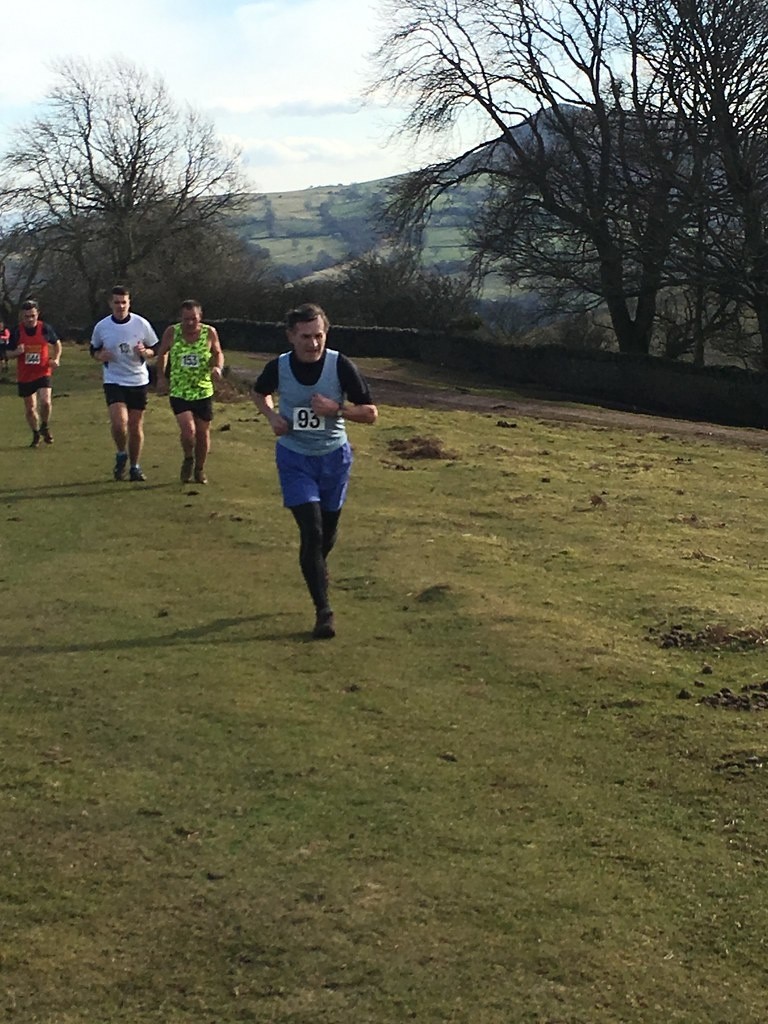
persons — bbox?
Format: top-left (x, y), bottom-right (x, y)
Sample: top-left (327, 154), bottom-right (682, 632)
top-left (252, 303), bottom-right (378, 638)
top-left (89, 286), bottom-right (160, 481)
top-left (6, 300), bottom-right (61, 449)
top-left (0, 321), bottom-right (10, 373)
top-left (156, 300), bottom-right (224, 485)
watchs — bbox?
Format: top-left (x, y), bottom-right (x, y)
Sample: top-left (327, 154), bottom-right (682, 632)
top-left (336, 403), bottom-right (343, 420)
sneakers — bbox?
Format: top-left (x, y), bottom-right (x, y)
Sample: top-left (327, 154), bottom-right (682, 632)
top-left (129, 467), bottom-right (148, 481)
top-left (313, 610), bottom-right (335, 636)
top-left (195, 468), bottom-right (208, 484)
top-left (113, 453), bottom-right (128, 482)
top-left (39, 428), bottom-right (54, 443)
top-left (180, 461), bottom-right (194, 482)
top-left (31, 435), bottom-right (41, 447)
